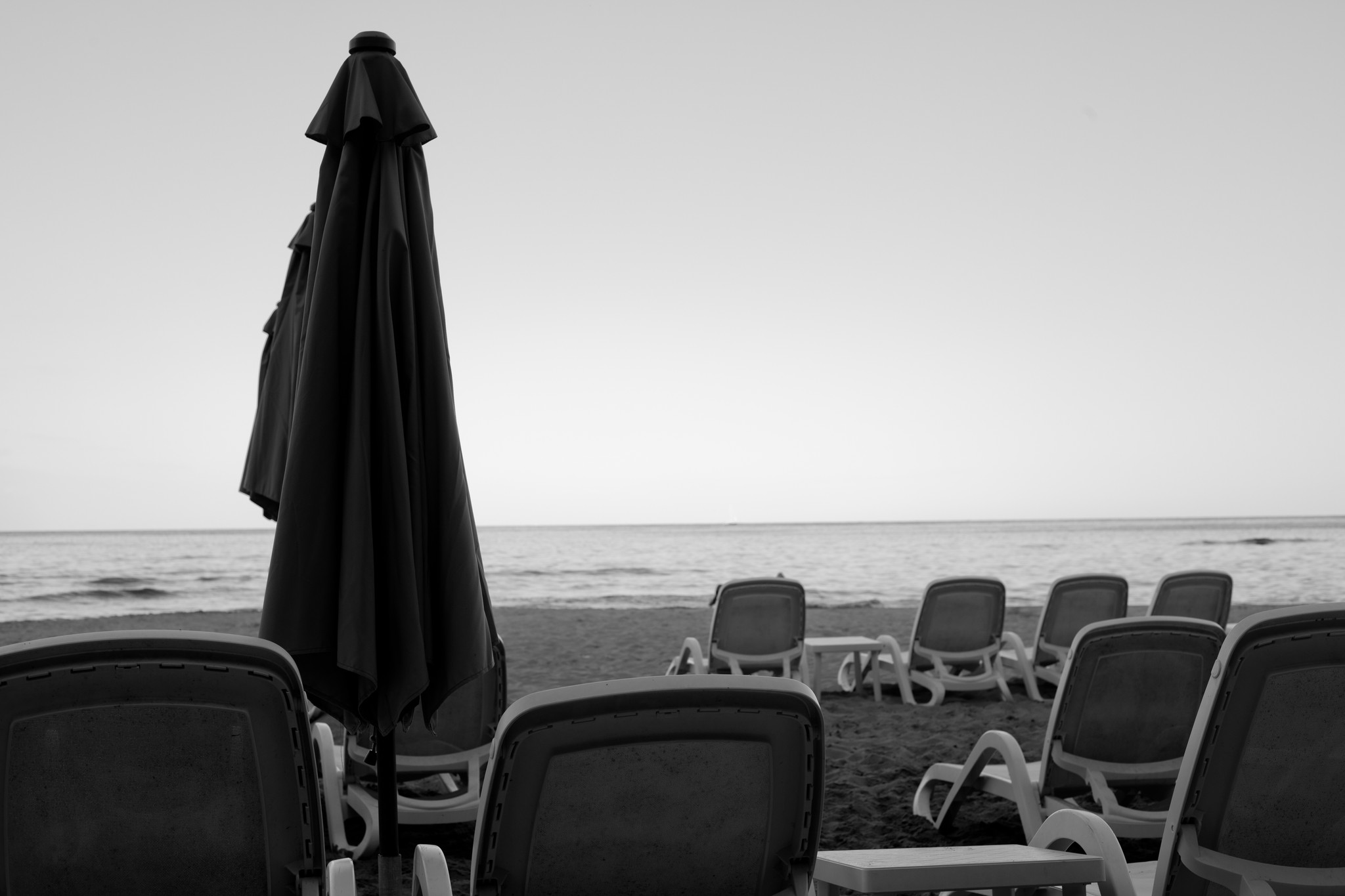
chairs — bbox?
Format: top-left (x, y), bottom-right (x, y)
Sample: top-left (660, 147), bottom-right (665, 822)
top-left (996, 573), bottom-right (1131, 703)
top-left (836, 575), bottom-right (1014, 705)
top-left (414, 674), bottom-right (828, 896)
top-left (1, 628), bottom-right (360, 896)
top-left (310, 632), bottom-right (515, 855)
top-left (913, 613), bottom-right (1229, 862)
top-left (664, 571), bottom-right (809, 680)
top-left (1143, 569), bottom-right (1233, 631)
top-left (1096, 605), bottom-right (1344, 895)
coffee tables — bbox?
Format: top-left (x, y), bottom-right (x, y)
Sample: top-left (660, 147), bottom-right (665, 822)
top-left (802, 637), bottom-right (886, 704)
top-left (814, 844), bottom-right (1104, 896)
